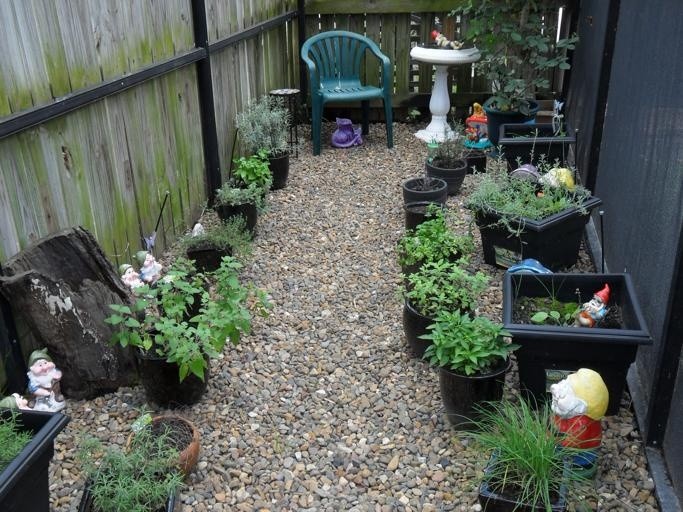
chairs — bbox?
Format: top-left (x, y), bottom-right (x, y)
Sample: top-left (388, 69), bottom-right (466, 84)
top-left (301, 30), bottom-right (393, 156)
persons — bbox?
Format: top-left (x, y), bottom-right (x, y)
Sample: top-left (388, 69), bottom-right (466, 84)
top-left (541, 368), bottom-right (609, 466)
top-left (432, 30), bottom-right (465, 51)
top-left (133, 249), bottom-right (172, 284)
top-left (0, 348), bottom-right (66, 414)
top-left (118, 262), bottom-right (146, 296)
top-left (576, 284), bottom-right (613, 327)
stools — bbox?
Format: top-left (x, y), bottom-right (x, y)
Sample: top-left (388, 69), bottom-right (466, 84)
top-left (270, 89), bottom-right (301, 159)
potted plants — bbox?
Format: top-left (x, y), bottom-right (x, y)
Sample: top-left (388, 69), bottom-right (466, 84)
top-left (213, 182), bottom-right (260, 229)
top-left (462, 131), bottom-right (601, 273)
top-left (231, 149), bottom-right (271, 200)
top-left (69, 408), bottom-right (196, 511)
top-left (398, 260), bottom-right (491, 357)
top-left (405, 164), bottom-right (448, 210)
top-left (498, 121), bottom-right (574, 167)
top-left (107, 257), bottom-right (276, 406)
top-left (122, 401), bottom-right (198, 476)
top-left (398, 203), bottom-right (473, 290)
top-left (501, 270), bottom-right (651, 417)
top-left (405, 203), bottom-right (447, 231)
top-left (427, 117), bottom-right (466, 195)
top-left (182, 216), bottom-right (252, 272)
top-left (445, 388), bottom-right (595, 512)
top-left (231, 93), bottom-right (293, 193)
top-left (0, 409), bottom-right (70, 511)
top-left (448, 0), bottom-right (580, 154)
top-left (464, 132), bottom-right (486, 173)
top-left (417, 308), bottom-right (510, 435)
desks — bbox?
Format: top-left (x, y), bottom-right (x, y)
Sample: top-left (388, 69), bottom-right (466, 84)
top-left (409, 42), bottom-right (480, 143)
